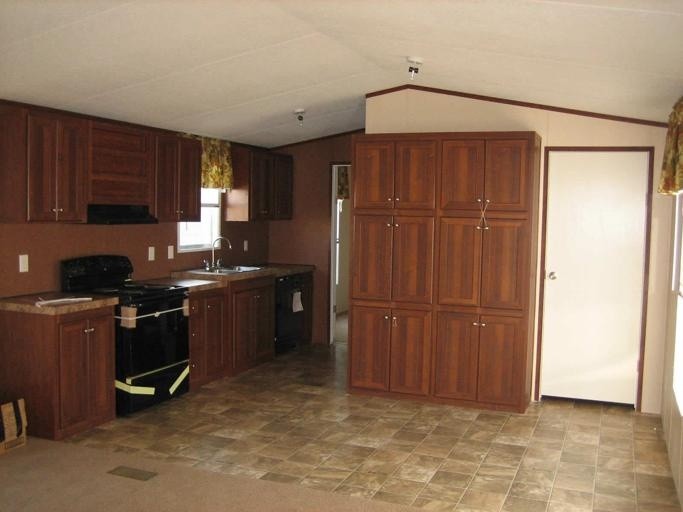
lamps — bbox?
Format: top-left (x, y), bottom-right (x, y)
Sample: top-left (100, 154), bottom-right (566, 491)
top-left (407, 56), bottom-right (423, 80)
top-left (293, 109), bottom-right (305, 128)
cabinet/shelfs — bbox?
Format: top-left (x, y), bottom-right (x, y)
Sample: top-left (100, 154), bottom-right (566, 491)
top-left (432, 132), bottom-right (541, 414)
top-left (0, 99), bottom-right (294, 225)
top-left (346, 132), bottom-right (434, 404)
top-left (1, 290), bottom-right (120, 442)
top-left (199, 275), bottom-right (276, 377)
top-left (135, 275), bottom-right (228, 389)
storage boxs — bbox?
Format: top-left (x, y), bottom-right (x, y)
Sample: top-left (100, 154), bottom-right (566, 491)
top-left (0, 399), bottom-right (27, 455)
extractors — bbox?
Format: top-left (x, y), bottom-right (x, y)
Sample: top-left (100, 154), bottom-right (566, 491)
top-left (86, 204), bottom-right (159, 225)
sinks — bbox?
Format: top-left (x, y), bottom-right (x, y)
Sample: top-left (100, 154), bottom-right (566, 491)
top-left (187, 268), bottom-right (235, 275)
top-left (216, 265), bottom-right (265, 272)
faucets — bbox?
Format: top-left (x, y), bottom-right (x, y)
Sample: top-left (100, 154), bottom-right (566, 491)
top-left (211, 237), bottom-right (234, 267)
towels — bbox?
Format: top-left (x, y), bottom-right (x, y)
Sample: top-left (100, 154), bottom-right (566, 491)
top-left (292, 292), bottom-right (303, 312)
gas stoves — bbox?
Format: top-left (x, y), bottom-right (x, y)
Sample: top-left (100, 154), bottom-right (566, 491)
top-left (74, 280), bottom-right (189, 301)
